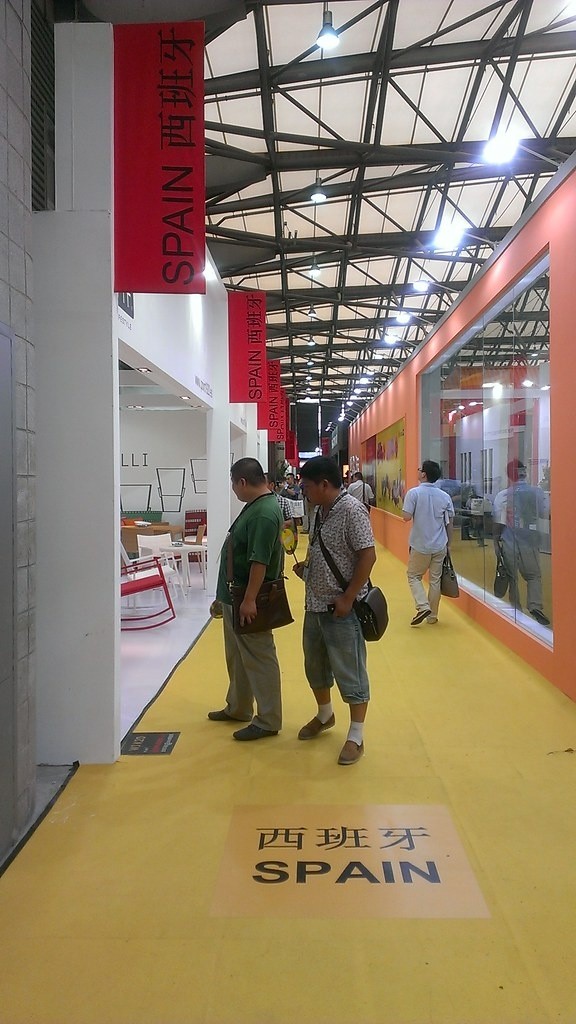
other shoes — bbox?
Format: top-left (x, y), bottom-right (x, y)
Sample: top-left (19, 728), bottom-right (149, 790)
top-left (530, 608), bottom-right (550, 625)
top-left (300, 530), bottom-right (309, 534)
top-left (428, 618), bottom-right (438, 625)
top-left (410, 608), bottom-right (432, 625)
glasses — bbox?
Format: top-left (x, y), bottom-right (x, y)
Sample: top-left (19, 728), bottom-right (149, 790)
top-left (287, 477), bottom-right (292, 481)
top-left (417, 468), bottom-right (422, 474)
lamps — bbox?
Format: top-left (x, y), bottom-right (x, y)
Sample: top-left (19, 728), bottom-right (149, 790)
top-left (304, 0), bottom-right (340, 396)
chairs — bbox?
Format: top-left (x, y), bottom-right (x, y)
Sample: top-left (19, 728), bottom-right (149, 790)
top-left (121, 515), bottom-right (209, 631)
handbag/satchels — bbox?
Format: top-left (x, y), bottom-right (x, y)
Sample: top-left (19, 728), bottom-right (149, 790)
top-left (356, 587), bottom-right (389, 641)
top-left (230, 577), bottom-right (295, 636)
top-left (440, 554), bottom-right (459, 598)
top-left (494, 551), bottom-right (509, 598)
top-left (362, 502), bottom-right (371, 514)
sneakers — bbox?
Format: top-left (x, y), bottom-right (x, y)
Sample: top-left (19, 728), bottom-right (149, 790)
top-left (298, 712), bottom-right (335, 740)
top-left (208, 709), bottom-right (245, 721)
top-left (338, 739), bottom-right (364, 765)
top-left (233, 723), bottom-right (279, 740)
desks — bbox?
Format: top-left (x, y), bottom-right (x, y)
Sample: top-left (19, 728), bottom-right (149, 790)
top-left (121, 525), bottom-right (186, 559)
top-left (159, 545), bottom-right (206, 592)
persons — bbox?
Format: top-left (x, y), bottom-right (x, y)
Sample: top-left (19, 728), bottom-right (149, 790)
top-left (291, 454), bottom-right (376, 766)
top-left (401, 460), bottom-right (455, 626)
top-left (207, 457), bottom-right (285, 740)
top-left (491, 460), bottom-right (550, 626)
top-left (271, 472), bottom-right (375, 534)
